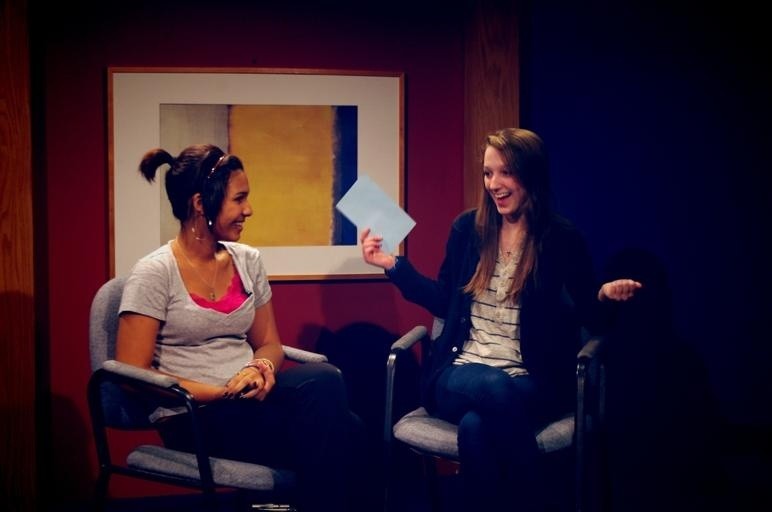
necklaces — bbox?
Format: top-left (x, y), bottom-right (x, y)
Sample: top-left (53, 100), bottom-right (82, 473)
top-left (176, 234), bottom-right (219, 302)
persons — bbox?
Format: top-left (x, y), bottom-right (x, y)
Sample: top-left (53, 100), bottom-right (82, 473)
top-left (114, 142), bottom-right (349, 509)
top-left (360, 126), bottom-right (643, 512)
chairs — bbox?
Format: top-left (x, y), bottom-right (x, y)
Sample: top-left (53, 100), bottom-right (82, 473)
top-left (367, 277), bottom-right (607, 510)
top-left (87, 275), bottom-right (349, 511)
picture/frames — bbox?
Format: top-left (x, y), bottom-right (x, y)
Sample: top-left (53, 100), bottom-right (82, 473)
top-left (107, 65), bottom-right (408, 283)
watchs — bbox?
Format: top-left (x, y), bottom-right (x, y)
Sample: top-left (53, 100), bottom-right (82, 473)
top-left (246, 359), bottom-right (277, 374)
